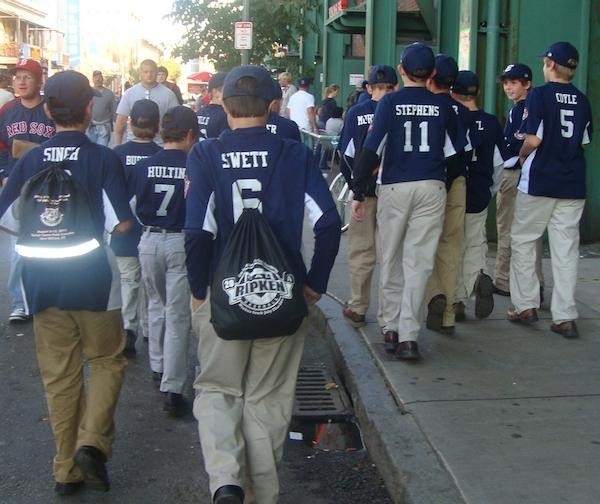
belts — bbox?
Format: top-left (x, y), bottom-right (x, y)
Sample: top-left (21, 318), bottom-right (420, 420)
top-left (143, 226), bottom-right (181, 233)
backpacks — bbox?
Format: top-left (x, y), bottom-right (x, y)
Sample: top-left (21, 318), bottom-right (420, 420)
top-left (211, 136), bottom-right (306, 340)
top-left (15, 141), bottom-right (101, 272)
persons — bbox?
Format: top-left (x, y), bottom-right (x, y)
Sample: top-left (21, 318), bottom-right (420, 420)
top-left (506, 42), bottom-right (593, 339)
top-left (0, 68), bottom-right (16, 110)
top-left (286, 78), bottom-right (320, 135)
top-left (156, 66), bottom-right (184, 106)
top-left (115, 59), bottom-right (180, 146)
top-left (0, 59), bottom-right (58, 325)
top-left (111, 98), bottom-right (160, 360)
top-left (86, 70), bottom-right (117, 147)
top-left (266, 76), bottom-right (303, 144)
top-left (195, 71), bottom-right (234, 144)
top-left (491, 63), bottom-right (545, 303)
top-left (351, 42), bottom-right (469, 361)
top-left (334, 65), bottom-right (400, 328)
top-left (314, 80), bottom-right (369, 170)
top-left (128, 105), bottom-right (207, 419)
top-left (184, 66), bottom-right (342, 504)
top-left (0, 70), bottom-right (133, 496)
top-left (425, 53), bottom-right (473, 337)
top-left (193, 85), bottom-right (211, 111)
top-left (278, 72), bottom-right (297, 121)
top-left (451, 70), bottom-right (506, 322)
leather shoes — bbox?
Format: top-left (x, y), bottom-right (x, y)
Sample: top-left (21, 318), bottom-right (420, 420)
top-left (384, 331), bottom-right (398, 351)
top-left (474, 273), bottom-right (494, 318)
top-left (125, 332), bottom-right (136, 359)
top-left (73, 447), bottom-right (109, 491)
top-left (427, 294), bottom-right (446, 330)
top-left (453, 302), bottom-right (464, 321)
top-left (506, 307), bottom-right (538, 323)
top-left (552, 321), bottom-right (577, 337)
top-left (55, 481), bottom-right (81, 495)
top-left (343, 308), bottom-right (365, 327)
top-left (395, 341), bottom-right (419, 359)
top-left (214, 486), bottom-right (243, 504)
top-left (164, 392), bottom-right (184, 413)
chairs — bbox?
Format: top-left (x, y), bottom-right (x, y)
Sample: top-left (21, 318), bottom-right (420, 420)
top-left (318, 129), bottom-right (339, 172)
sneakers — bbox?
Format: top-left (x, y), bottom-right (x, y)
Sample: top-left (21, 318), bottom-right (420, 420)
top-left (9, 308), bottom-right (29, 322)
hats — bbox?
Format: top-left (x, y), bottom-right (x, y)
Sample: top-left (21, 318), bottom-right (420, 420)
top-left (536, 42), bottom-right (578, 69)
top-left (131, 99), bottom-right (159, 128)
top-left (400, 42), bottom-right (435, 77)
top-left (434, 54), bottom-right (458, 84)
top-left (162, 106), bottom-right (205, 137)
top-left (368, 65), bottom-right (398, 84)
top-left (223, 65), bottom-right (282, 100)
top-left (11, 58), bottom-right (42, 75)
top-left (454, 70), bottom-right (480, 95)
top-left (499, 63), bottom-right (532, 80)
top-left (46, 71), bottom-right (101, 112)
